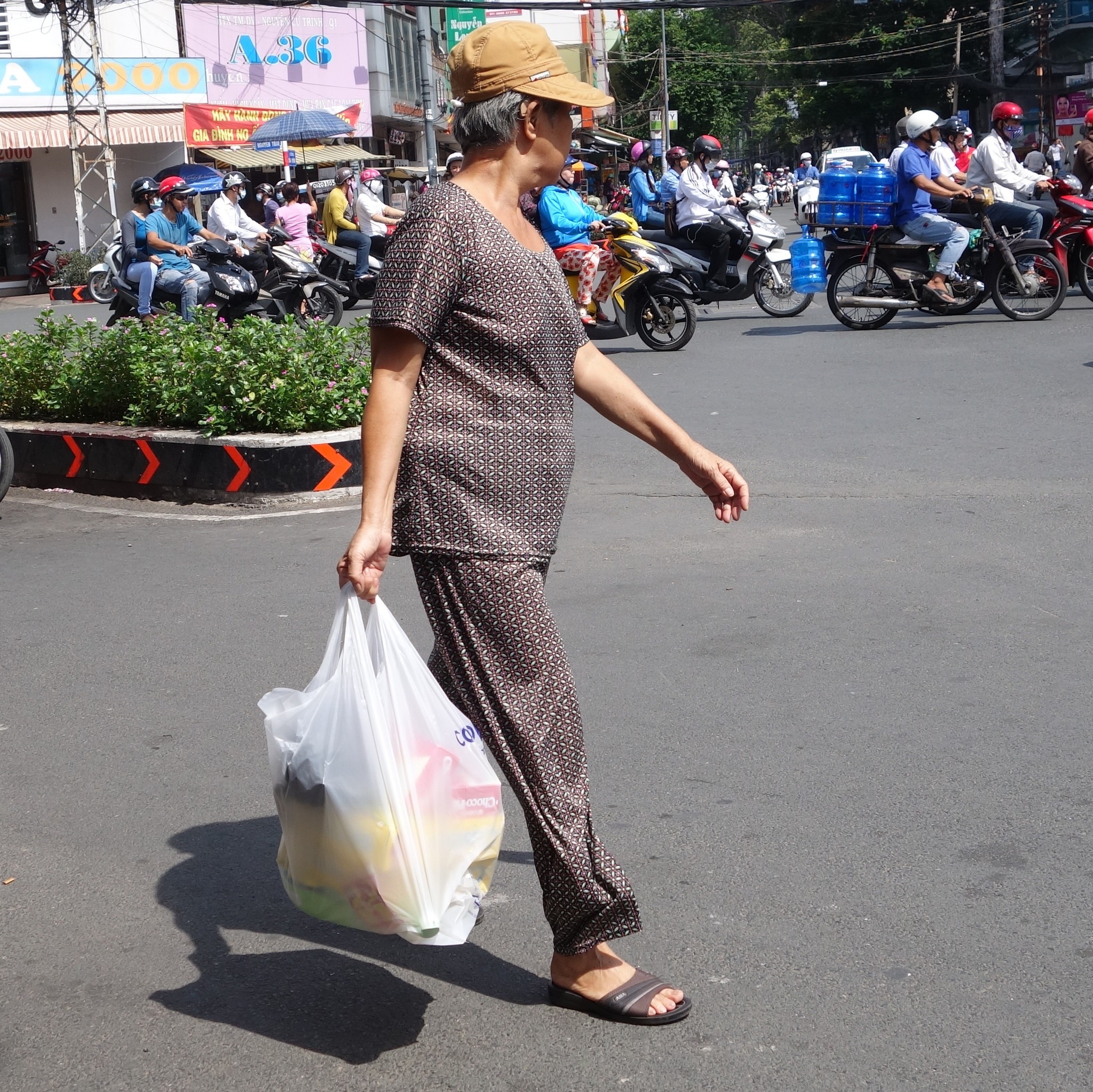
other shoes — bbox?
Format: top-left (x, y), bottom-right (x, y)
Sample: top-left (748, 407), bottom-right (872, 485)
top-left (140, 314), bottom-right (156, 322)
top-left (577, 303), bottom-right (597, 325)
top-left (354, 274), bottom-right (374, 279)
top-left (591, 296), bottom-right (610, 321)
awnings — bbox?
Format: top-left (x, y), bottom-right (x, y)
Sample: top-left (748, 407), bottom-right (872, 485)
top-left (573, 124), bottom-right (642, 152)
top-left (199, 141), bottom-right (396, 170)
top-left (0, 112), bottom-right (186, 152)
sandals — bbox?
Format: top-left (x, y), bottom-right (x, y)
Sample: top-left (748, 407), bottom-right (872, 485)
top-left (1033, 273), bottom-right (1048, 285)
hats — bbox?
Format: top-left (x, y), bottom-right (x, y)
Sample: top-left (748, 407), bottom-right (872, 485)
top-left (238, 184), bottom-right (245, 190)
top-left (446, 20), bottom-right (615, 107)
top-left (1075, 140), bottom-right (1081, 146)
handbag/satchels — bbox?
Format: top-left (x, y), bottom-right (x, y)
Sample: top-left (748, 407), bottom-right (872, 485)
top-left (1044, 150), bottom-right (1054, 165)
top-left (258, 582), bottom-right (505, 946)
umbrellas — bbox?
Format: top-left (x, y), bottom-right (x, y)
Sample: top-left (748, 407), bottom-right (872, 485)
top-left (246, 103), bottom-right (357, 181)
top-left (381, 164), bottom-right (448, 191)
top-left (153, 161), bottom-right (225, 218)
top-left (571, 159), bottom-right (599, 197)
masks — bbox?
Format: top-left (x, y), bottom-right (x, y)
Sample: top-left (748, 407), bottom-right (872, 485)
top-left (963, 140), bottom-right (967, 146)
top-left (779, 172), bottom-right (784, 175)
top-left (236, 189), bottom-right (246, 200)
top-left (256, 194), bottom-right (262, 201)
top-left (804, 160), bottom-right (811, 167)
top-left (1003, 125), bottom-right (1023, 139)
top-left (276, 191), bottom-right (284, 200)
top-left (755, 170), bottom-right (761, 175)
top-left (349, 182), bottom-right (356, 190)
top-left (714, 170), bottom-right (722, 177)
top-left (150, 198), bottom-right (165, 209)
top-left (705, 158), bottom-right (718, 170)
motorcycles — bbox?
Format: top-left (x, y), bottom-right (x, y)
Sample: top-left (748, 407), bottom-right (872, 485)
top-left (24, 147), bottom-right (1092, 351)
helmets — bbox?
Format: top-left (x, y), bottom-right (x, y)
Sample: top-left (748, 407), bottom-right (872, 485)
top-left (906, 110), bottom-right (947, 138)
top-left (694, 135), bottom-right (722, 164)
top-left (763, 165), bottom-right (789, 172)
top-left (274, 180), bottom-right (288, 189)
top-left (608, 174), bottom-right (614, 179)
top-left (800, 152), bottom-right (812, 160)
top-left (963, 127), bottom-right (972, 140)
top-left (631, 140), bottom-right (652, 161)
top-left (716, 160), bottom-right (729, 169)
top-left (1032, 143), bottom-right (1039, 149)
top-left (939, 119), bottom-right (968, 139)
top-left (991, 101), bottom-right (1026, 122)
top-left (1085, 109), bottom-right (1093, 127)
top-left (565, 154), bottom-right (579, 165)
top-left (222, 171), bottom-right (251, 191)
top-left (334, 167), bottom-right (355, 184)
top-left (666, 146), bottom-right (691, 166)
top-left (361, 169), bottom-right (386, 183)
top-left (159, 176), bottom-right (195, 200)
top-left (255, 183), bottom-right (275, 196)
top-left (754, 163), bottom-right (762, 169)
top-left (896, 116), bottom-right (911, 136)
top-left (131, 176), bottom-right (161, 202)
top-left (446, 152), bottom-right (463, 170)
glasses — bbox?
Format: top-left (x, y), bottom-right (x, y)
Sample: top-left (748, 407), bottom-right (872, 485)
top-left (450, 166), bottom-right (461, 171)
top-left (257, 191), bottom-right (262, 193)
top-left (173, 195), bottom-right (188, 201)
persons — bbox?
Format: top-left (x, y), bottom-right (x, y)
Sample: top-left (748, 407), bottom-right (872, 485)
top-left (1073, 140), bottom-right (1082, 158)
top-left (628, 141), bottom-right (665, 230)
top-left (712, 159), bottom-right (736, 200)
top-left (274, 180), bottom-right (289, 207)
top-left (519, 187), bottom-right (541, 233)
top-left (322, 167), bottom-right (376, 279)
top-left (357, 168), bottom-right (405, 257)
top-left (1055, 94), bottom-right (1077, 120)
top-left (602, 176), bottom-right (619, 205)
top-left (446, 152), bottom-right (464, 177)
top-left (274, 179), bottom-right (320, 321)
top-left (207, 171), bottom-right (274, 304)
top-left (1046, 137), bottom-right (1065, 177)
top-left (661, 147), bottom-right (692, 208)
top-left (897, 109), bottom-right (980, 304)
top-left (539, 153), bottom-right (622, 325)
top-left (732, 152), bottom-right (821, 216)
top-left (253, 183), bottom-right (280, 230)
top-left (334, 17), bottom-right (749, 1025)
top-left (584, 171), bottom-right (598, 195)
top-left (1023, 143), bottom-right (1048, 175)
top-left (144, 176), bottom-right (243, 325)
top-left (887, 101), bottom-right (977, 196)
top-left (119, 176), bottom-right (160, 324)
top-left (853, 157), bottom-right (869, 170)
top-left (1072, 108), bottom-right (1093, 199)
top-left (964, 101), bottom-right (1055, 285)
top-left (676, 135), bottom-right (745, 293)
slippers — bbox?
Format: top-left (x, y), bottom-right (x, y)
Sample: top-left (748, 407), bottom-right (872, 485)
top-left (923, 284), bottom-right (958, 304)
top-left (548, 961), bottom-right (692, 1026)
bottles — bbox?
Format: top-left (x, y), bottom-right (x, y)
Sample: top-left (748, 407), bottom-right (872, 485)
top-left (789, 225), bottom-right (828, 293)
top-left (818, 159), bottom-right (898, 225)
